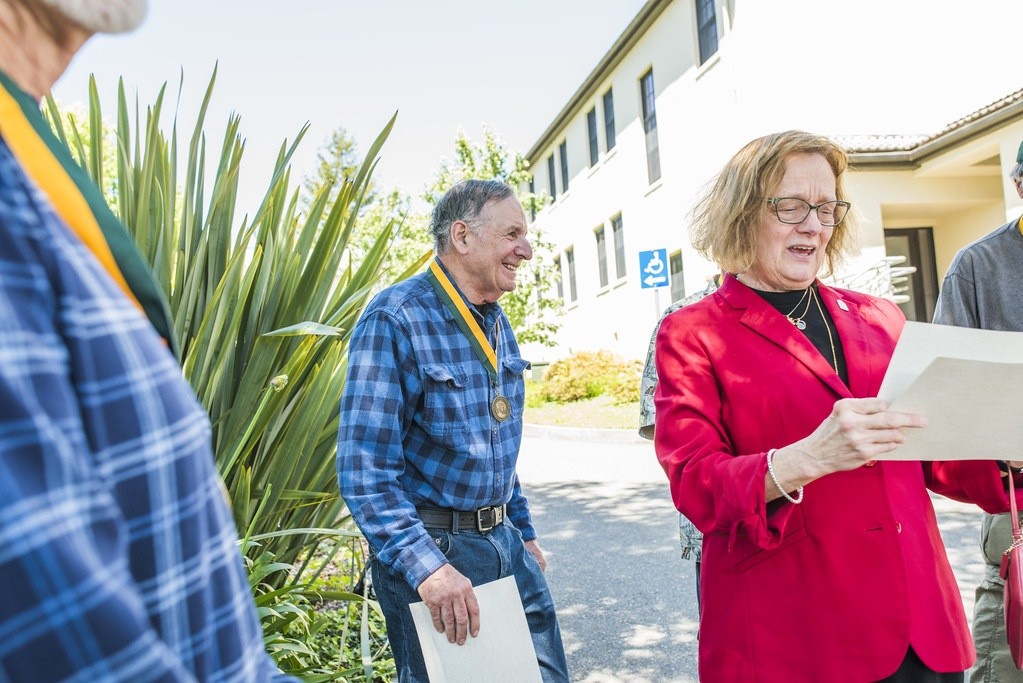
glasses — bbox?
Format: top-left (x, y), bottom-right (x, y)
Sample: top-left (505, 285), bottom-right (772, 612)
top-left (764, 197), bottom-right (852, 226)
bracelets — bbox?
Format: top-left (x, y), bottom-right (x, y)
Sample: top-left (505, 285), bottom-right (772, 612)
top-left (766, 449), bottom-right (803, 504)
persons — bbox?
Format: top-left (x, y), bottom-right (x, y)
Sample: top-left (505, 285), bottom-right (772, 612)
top-left (931, 140), bottom-right (1023, 683)
top-left (335, 180), bottom-right (568, 683)
top-left (652, 132), bottom-right (1023, 683)
top-left (1, 0), bottom-right (304, 683)
top-left (637, 265), bottom-right (726, 618)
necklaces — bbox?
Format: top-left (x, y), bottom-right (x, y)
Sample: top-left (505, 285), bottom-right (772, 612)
top-left (783, 286), bottom-right (839, 376)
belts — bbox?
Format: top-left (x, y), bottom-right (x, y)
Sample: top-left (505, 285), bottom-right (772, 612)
top-left (414, 504), bottom-right (506, 533)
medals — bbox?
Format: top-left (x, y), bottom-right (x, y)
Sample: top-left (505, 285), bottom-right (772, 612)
top-left (492, 396), bottom-right (511, 422)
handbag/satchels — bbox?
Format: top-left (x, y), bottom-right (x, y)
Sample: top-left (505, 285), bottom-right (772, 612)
top-left (1001, 465), bottom-right (1023, 671)
